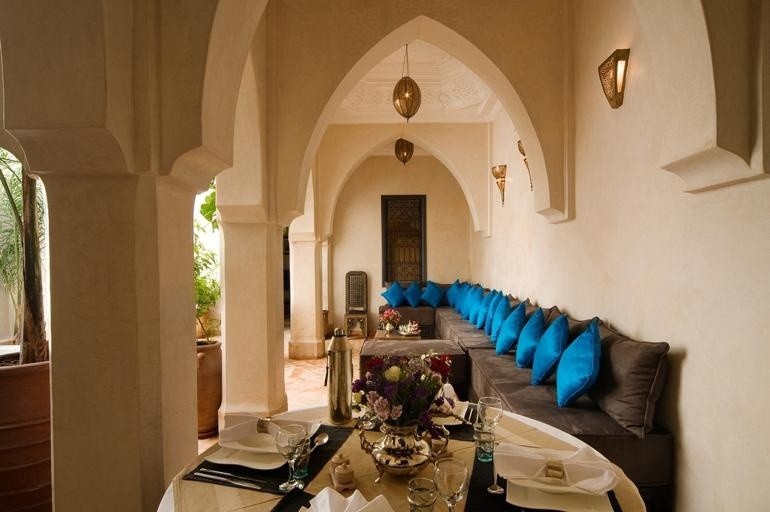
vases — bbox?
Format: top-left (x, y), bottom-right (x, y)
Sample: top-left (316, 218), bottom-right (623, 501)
top-left (357, 422), bottom-right (453, 484)
top-left (383, 322), bottom-right (395, 336)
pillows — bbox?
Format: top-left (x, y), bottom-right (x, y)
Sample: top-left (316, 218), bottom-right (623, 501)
top-left (381, 281), bottom-right (405, 307)
top-left (403, 281), bottom-right (423, 308)
top-left (483, 288), bottom-right (598, 345)
top-left (456, 281), bottom-right (503, 335)
top-left (490, 295), bottom-right (511, 343)
top-left (515, 307), bottom-right (545, 368)
top-left (495, 301), bottom-right (527, 353)
top-left (531, 314), bottom-right (570, 385)
top-left (556, 318), bottom-right (602, 407)
top-left (585, 317), bottom-right (671, 440)
top-left (447, 278), bottom-right (461, 308)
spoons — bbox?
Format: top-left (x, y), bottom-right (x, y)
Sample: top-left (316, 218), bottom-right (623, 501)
top-left (465, 408), bottom-right (473, 425)
top-left (296, 433), bottom-right (329, 465)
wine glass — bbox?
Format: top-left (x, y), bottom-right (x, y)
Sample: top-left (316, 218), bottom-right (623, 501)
top-left (477, 397), bottom-right (503, 430)
top-left (433, 458), bottom-right (470, 512)
top-left (275, 424), bottom-right (306, 493)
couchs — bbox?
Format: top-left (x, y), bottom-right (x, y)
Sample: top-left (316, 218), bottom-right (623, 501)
top-left (359, 340), bottom-right (466, 384)
top-left (380, 306), bottom-right (436, 338)
top-left (436, 306), bottom-right (499, 349)
top-left (467, 349), bottom-right (676, 512)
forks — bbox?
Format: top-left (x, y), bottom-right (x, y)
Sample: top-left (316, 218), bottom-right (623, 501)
top-left (473, 415), bottom-right (481, 428)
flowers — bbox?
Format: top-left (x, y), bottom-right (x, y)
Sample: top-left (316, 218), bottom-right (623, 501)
top-left (351, 350), bottom-right (472, 441)
top-left (379, 309), bottom-right (401, 328)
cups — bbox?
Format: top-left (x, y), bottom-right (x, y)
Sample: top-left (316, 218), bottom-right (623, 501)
top-left (407, 478), bottom-right (437, 512)
top-left (330, 452), bottom-right (350, 471)
top-left (335, 462), bottom-right (354, 484)
top-left (289, 438), bottom-right (311, 478)
top-left (473, 423), bottom-right (495, 462)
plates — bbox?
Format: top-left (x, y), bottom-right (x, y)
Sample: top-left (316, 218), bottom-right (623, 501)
top-left (203, 417), bottom-right (324, 470)
top-left (430, 401), bottom-right (469, 426)
top-left (505, 479), bottom-right (615, 512)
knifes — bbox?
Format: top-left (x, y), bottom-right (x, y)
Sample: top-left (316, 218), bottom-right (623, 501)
top-left (194, 472), bottom-right (262, 490)
top-left (200, 468), bottom-right (267, 483)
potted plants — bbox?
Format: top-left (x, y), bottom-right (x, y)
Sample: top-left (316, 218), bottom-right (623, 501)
top-left (0, 149), bottom-right (52, 512)
top-left (193, 216), bottom-right (221, 439)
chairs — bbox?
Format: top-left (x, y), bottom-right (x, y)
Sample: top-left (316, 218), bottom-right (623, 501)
top-left (421, 280), bottom-right (447, 307)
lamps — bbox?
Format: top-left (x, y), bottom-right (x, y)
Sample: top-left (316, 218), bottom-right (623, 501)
top-left (492, 165), bottom-right (506, 208)
top-left (393, 43), bottom-right (422, 121)
top-left (598, 48), bottom-right (630, 109)
